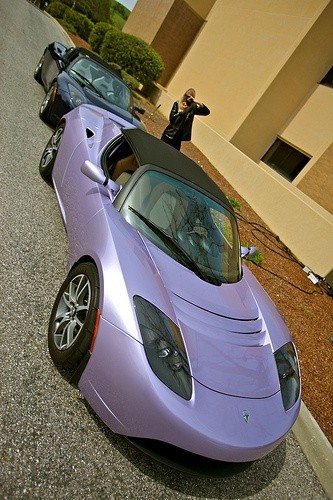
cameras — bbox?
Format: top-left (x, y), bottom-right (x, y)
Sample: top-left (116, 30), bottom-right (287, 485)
top-left (185, 96), bottom-right (193, 106)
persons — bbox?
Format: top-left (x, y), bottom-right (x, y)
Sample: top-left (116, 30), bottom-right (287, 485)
top-left (161, 88), bottom-right (210, 151)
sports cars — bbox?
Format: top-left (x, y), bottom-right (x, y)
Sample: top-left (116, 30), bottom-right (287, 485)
top-left (33, 41), bottom-right (148, 133)
top-left (39, 103), bottom-right (303, 478)
